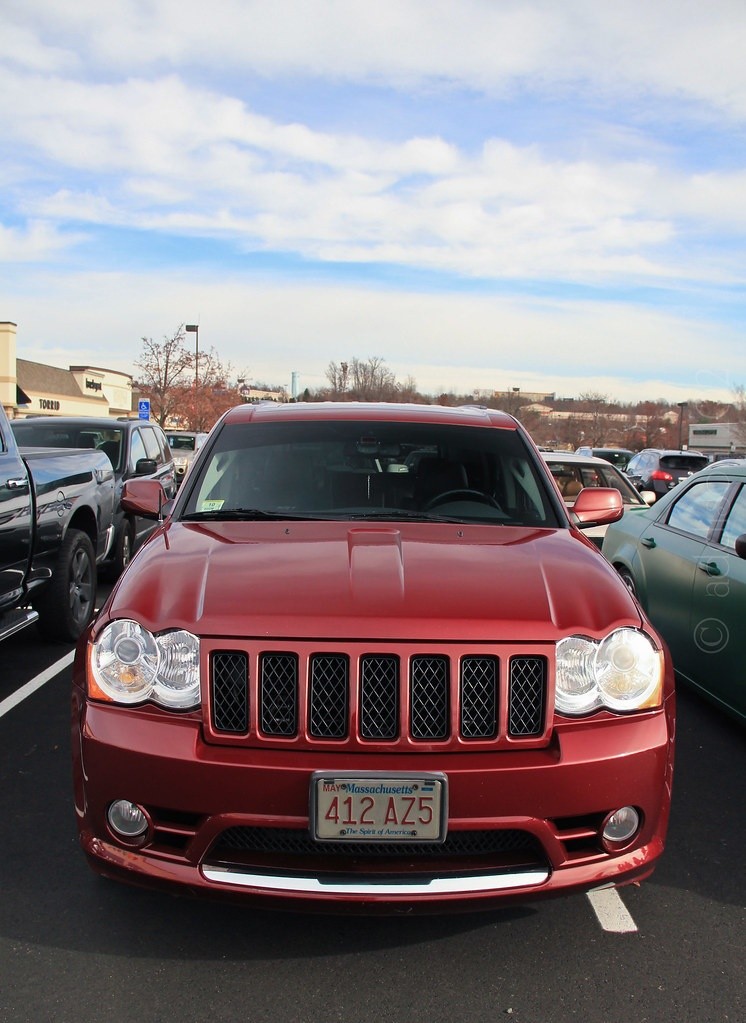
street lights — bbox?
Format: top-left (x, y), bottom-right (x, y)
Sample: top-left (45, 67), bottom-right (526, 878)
top-left (184, 323), bottom-right (198, 390)
top-left (512, 388), bottom-right (520, 398)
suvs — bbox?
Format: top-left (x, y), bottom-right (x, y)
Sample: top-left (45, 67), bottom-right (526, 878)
top-left (66, 398), bottom-right (678, 917)
top-left (622, 446), bottom-right (711, 504)
top-left (0, 404), bottom-right (118, 651)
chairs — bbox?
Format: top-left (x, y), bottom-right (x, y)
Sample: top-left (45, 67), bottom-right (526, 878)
top-left (93, 441), bottom-right (121, 472)
top-left (561, 479), bottom-right (584, 502)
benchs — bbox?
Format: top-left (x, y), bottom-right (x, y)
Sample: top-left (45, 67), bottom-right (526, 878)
top-left (331, 468), bottom-right (414, 510)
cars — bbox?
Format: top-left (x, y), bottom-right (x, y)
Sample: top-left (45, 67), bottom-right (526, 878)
top-left (6, 415), bottom-right (177, 578)
top-left (597, 458), bottom-right (745, 734)
top-left (162, 430), bottom-right (214, 483)
top-left (572, 445), bottom-right (637, 471)
top-left (537, 452), bottom-right (658, 548)
